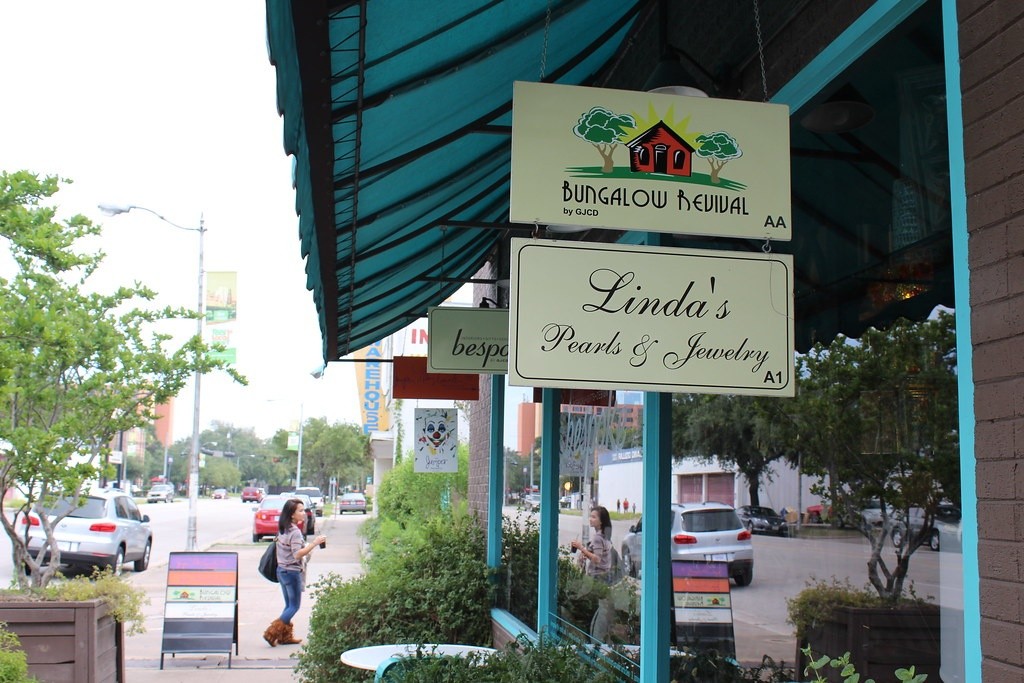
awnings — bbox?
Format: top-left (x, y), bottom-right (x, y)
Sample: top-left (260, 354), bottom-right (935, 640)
top-left (265, 0), bottom-right (655, 378)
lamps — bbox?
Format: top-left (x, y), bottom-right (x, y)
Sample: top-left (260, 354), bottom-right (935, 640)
top-left (859, 176), bottom-right (934, 307)
top-left (798, 82), bottom-right (876, 134)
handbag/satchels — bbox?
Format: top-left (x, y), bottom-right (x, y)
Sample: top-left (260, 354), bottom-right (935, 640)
top-left (605, 545), bottom-right (630, 588)
top-left (259, 532), bottom-right (279, 583)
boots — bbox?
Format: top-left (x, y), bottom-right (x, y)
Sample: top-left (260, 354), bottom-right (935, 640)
top-left (263, 619), bottom-right (286, 647)
top-left (278, 623), bottom-right (302, 645)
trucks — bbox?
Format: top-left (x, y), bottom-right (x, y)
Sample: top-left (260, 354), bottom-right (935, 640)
top-left (151, 478), bottom-right (174, 488)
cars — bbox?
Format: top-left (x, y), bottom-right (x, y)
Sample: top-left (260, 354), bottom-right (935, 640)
top-left (734, 506), bottom-right (788, 537)
top-left (854, 499), bottom-right (891, 525)
top-left (212, 489), bottom-right (228, 499)
top-left (252, 497), bottom-right (307, 542)
top-left (891, 502), bottom-right (961, 551)
top-left (279, 492), bottom-right (316, 534)
top-left (147, 484), bottom-right (174, 503)
top-left (524, 495), bottom-right (540, 511)
top-left (258, 488), bottom-right (266, 498)
top-left (559, 497), bottom-right (571, 508)
top-left (340, 492), bottom-right (366, 514)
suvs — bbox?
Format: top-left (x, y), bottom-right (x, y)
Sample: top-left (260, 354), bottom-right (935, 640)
top-left (241, 487), bottom-right (262, 503)
top-left (621, 502), bottom-right (753, 587)
top-left (13, 489), bottom-right (154, 578)
top-left (104, 481), bottom-right (141, 496)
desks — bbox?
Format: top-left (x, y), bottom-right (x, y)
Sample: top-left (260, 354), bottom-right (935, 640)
top-left (555, 642), bottom-right (700, 662)
top-left (340, 640), bottom-right (508, 670)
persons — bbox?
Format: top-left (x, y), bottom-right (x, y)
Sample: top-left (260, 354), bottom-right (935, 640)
top-left (572, 506), bottom-right (613, 644)
top-left (263, 500), bottom-right (326, 646)
top-left (617, 498), bottom-right (636, 514)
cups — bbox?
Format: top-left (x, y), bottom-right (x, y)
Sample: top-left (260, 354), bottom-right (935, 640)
top-left (320, 535), bottom-right (326, 549)
top-left (571, 541), bottom-right (577, 553)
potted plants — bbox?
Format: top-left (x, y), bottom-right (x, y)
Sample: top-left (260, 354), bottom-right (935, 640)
top-left (0, 170), bottom-right (238, 683)
top-left (772, 315), bottom-right (962, 683)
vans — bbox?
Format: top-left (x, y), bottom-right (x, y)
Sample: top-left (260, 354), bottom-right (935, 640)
top-left (294, 487), bottom-right (324, 517)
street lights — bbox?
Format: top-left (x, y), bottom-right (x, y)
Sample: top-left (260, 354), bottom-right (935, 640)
top-left (97, 200), bottom-right (206, 549)
top-left (268, 398), bottom-right (305, 491)
